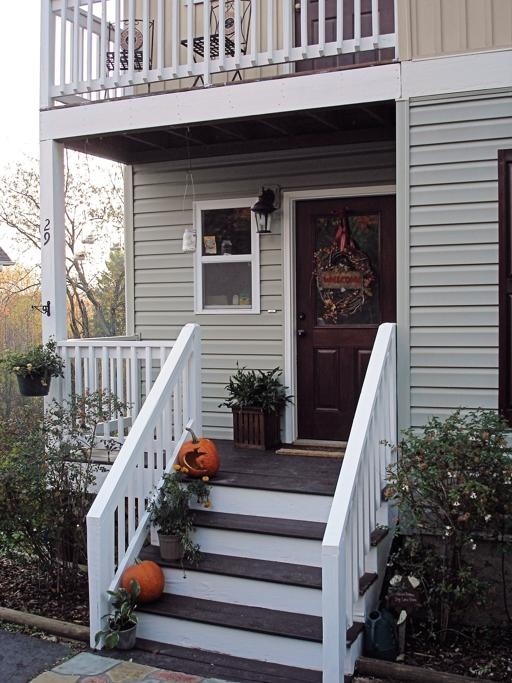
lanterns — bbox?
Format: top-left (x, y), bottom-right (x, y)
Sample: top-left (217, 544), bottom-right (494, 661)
top-left (177, 423), bottom-right (221, 476)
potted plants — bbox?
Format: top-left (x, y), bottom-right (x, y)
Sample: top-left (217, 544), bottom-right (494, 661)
top-left (93, 576), bottom-right (141, 649)
top-left (217, 359), bottom-right (296, 451)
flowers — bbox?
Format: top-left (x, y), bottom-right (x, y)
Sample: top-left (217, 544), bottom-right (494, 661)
top-left (0, 334), bottom-right (66, 387)
top-left (143, 464), bottom-right (213, 578)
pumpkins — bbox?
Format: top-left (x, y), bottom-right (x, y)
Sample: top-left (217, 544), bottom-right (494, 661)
top-left (121, 556), bottom-right (165, 604)
top-left (179, 428), bottom-right (220, 478)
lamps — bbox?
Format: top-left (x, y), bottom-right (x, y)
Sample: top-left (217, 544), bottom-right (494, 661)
top-left (251, 187), bottom-right (278, 233)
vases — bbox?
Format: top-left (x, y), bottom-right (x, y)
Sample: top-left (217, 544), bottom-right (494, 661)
top-left (157, 529), bottom-right (185, 561)
top-left (16, 373), bottom-right (50, 395)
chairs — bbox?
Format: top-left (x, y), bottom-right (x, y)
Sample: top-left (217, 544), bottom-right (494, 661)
top-left (103, 0), bottom-right (252, 100)
